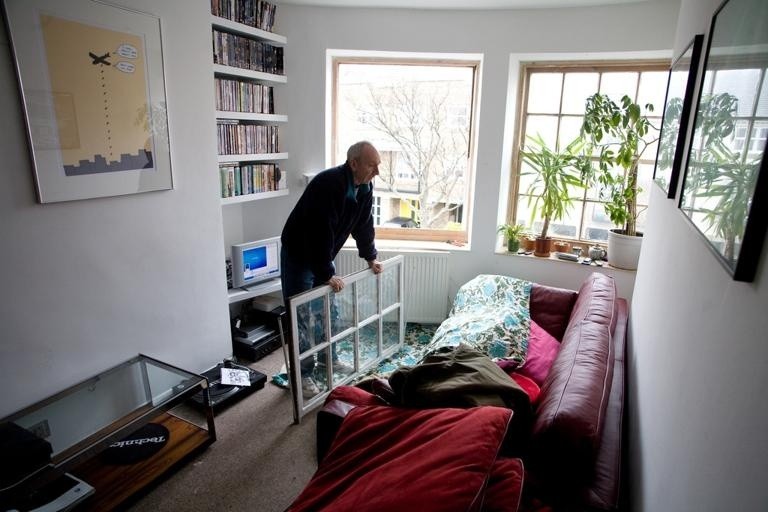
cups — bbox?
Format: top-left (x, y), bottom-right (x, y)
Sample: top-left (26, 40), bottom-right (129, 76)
top-left (573, 247), bottom-right (582, 257)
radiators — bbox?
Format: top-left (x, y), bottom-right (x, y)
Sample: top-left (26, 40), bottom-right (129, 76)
top-left (331, 245), bottom-right (453, 328)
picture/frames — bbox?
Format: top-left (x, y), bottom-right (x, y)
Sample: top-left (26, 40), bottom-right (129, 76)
top-left (671, 0), bottom-right (768, 282)
top-left (650, 31), bottom-right (707, 200)
top-left (0, 0), bottom-right (180, 206)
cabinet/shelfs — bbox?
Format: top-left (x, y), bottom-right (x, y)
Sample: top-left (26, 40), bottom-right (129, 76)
top-left (212, 10), bottom-right (293, 208)
top-left (0, 351), bottom-right (216, 511)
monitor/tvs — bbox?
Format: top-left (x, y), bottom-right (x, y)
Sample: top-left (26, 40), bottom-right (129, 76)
top-left (231, 235), bottom-right (282, 291)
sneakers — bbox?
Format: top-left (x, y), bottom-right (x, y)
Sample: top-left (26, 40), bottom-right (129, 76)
top-left (302, 375), bottom-right (320, 397)
top-left (318, 358), bottom-right (355, 374)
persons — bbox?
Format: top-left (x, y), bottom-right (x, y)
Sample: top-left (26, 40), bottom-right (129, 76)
top-left (281, 141), bottom-right (382, 398)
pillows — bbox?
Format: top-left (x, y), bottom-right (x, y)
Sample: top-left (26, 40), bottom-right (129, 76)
top-left (505, 367), bottom-right (541, 404)
top-left (519, 313), bottom-right (560, 388)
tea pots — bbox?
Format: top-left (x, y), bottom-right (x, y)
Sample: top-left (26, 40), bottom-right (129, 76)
top-left (586, 243), bottom-right (607, 259)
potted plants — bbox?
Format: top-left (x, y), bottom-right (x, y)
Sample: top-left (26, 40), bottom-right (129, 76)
top-left (514, 127), bottom-right (594, 258)
top-left (495, 215), bottom-right (525, 255)
top-left (578, 92), bottom-right (663, 272)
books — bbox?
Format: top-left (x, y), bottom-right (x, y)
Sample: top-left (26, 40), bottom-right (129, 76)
top-left (213, 0), bottom-right (285, 199)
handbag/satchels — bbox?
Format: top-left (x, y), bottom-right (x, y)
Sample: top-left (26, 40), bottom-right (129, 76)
top-left (388, 342), bottom-right (529, 407)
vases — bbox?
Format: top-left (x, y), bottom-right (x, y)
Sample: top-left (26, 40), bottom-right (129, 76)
top-left (521, 234), bottom-right (537, 254)
top-left (553, 241), bottom-right (571, 256)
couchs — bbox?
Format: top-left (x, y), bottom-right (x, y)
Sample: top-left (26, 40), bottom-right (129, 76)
top-left (308, 267), bottom-right (643, 511)
